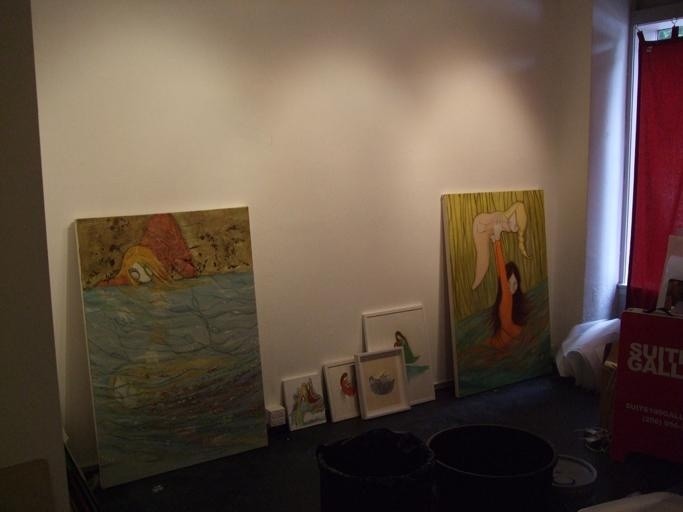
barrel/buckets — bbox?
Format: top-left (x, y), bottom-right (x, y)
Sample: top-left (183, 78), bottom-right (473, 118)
top-left (314, 431), bottom-right (438, 512)
top-left (554, 455), bottom-right (598, 512)
top-left (426, 421), bottom-right (559, 511)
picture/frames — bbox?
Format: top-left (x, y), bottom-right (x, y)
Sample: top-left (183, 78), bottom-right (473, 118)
top-left (322, 303), bottom-right (437, 424)
